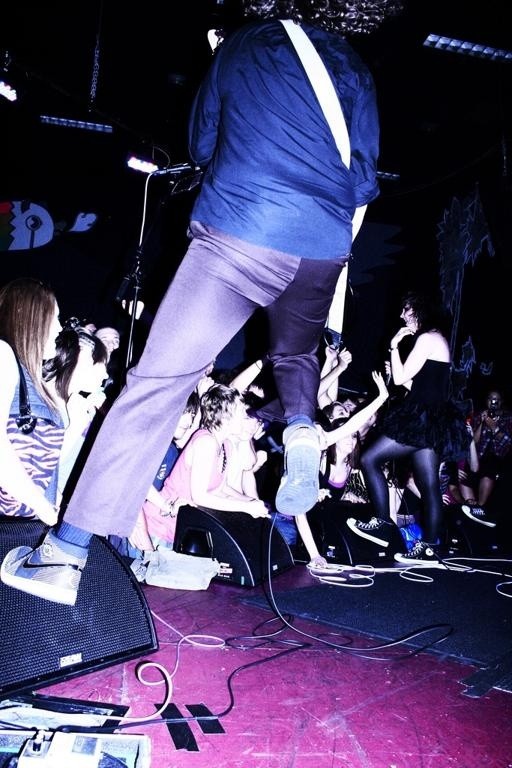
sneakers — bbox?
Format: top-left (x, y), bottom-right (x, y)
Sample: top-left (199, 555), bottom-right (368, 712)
top-left (276, 426), bottom-right (321, 515)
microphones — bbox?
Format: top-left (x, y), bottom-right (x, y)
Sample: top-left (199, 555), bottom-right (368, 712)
top-left (116, 276), bottom-right (131, 301)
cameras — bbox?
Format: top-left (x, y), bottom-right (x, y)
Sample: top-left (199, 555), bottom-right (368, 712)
top-left (488, 399), bottom-right (499, 418)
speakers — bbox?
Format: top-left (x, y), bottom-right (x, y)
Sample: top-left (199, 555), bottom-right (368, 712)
top-left (308, 499), bottom-right (410, 563)
top-left (0, 514), bottom-right (160, 704)
top-left (173, 504), bottom-right (294, 588)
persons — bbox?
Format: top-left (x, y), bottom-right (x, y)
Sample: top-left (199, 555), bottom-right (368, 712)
top-left (4, 18), bottom-right (383, 609)
top-left (0, 269), bottom-right (511, 580)
